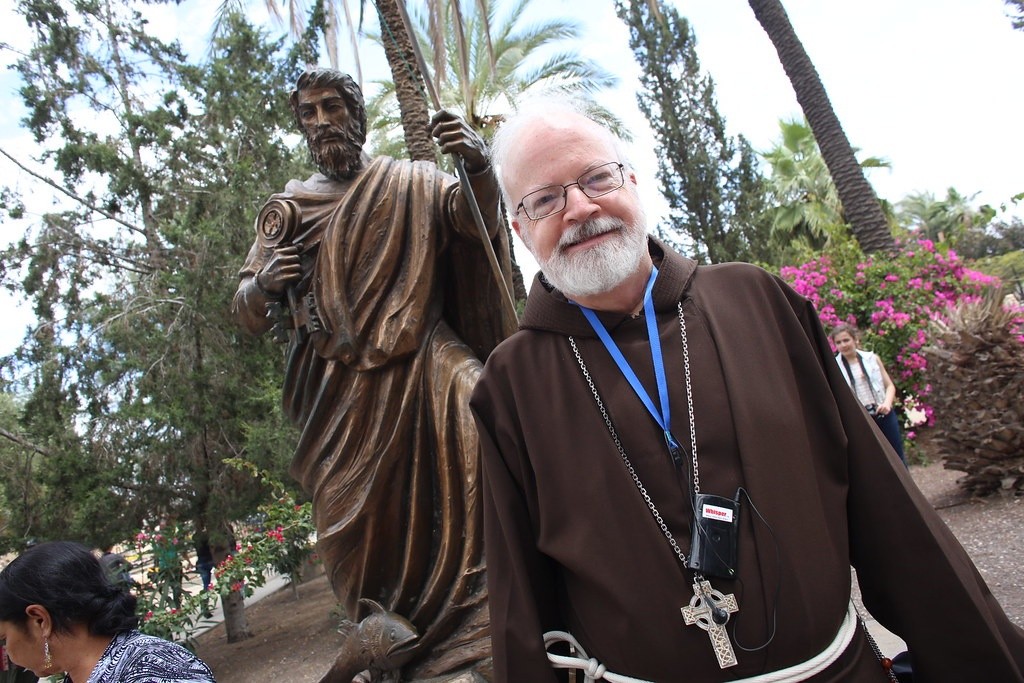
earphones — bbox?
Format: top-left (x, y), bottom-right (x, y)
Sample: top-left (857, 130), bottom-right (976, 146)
top-left (705, 595), bottom-right (729, 625)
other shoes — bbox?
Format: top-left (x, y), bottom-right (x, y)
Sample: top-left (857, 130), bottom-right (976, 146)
top-left (203, 611), bottom-right (213, 619)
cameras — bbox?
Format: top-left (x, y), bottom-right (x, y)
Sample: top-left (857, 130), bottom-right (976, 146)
top-left (864, 403), bottom-right (883, 423)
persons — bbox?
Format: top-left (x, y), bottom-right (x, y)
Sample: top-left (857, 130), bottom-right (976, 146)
top-left (0, 517), bottom-right (236, 682)
top-left (230, 68), bottom-right (521, 683)
top-left (834, 325), bottom-right (905, 467)
top-left (468, 95), bottom-right (1024, 682)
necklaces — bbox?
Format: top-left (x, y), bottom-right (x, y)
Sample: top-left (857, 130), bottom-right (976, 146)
top-left (566, 302), bottom-right (742, 671)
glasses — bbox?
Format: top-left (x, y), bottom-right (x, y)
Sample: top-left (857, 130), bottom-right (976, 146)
top-left (516, 160), bottom-right (624, 221)
